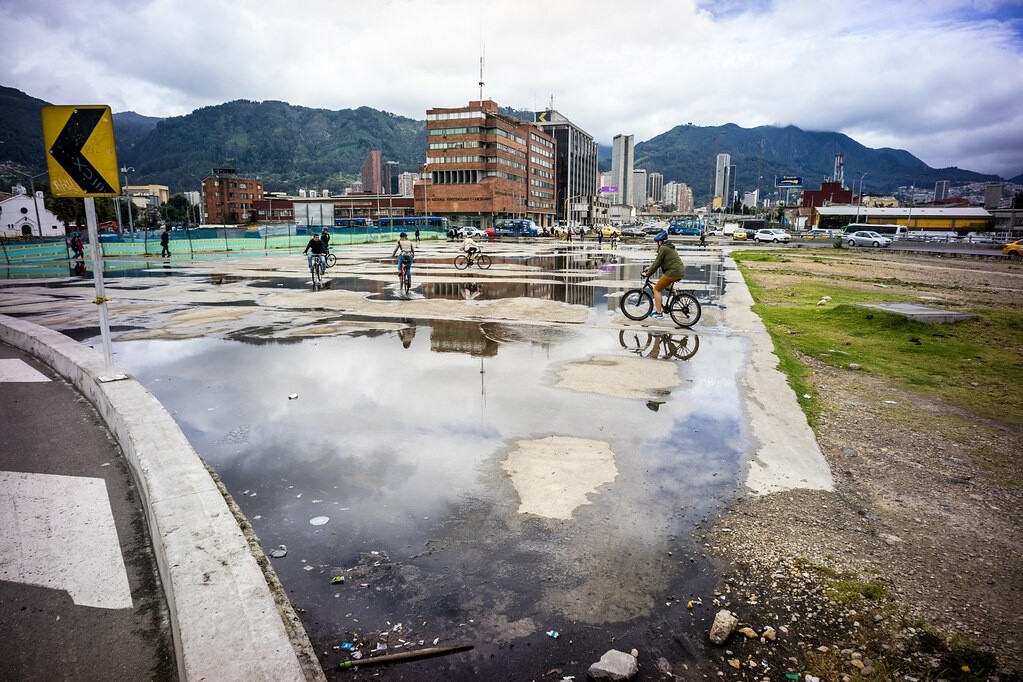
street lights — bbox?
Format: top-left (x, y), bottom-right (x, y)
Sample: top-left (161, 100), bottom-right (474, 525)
top-left (903, 174), bottom-right (916, 227)
top-left (856, 172), bottom-right (868, 224)
top-left (120, 162), bottom-right (136, 233)
top-left (563, 195), bottom-right (581, 227)
top-left (192, 174), bottom-right (212, 225)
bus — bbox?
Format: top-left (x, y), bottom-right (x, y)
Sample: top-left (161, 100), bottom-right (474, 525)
top-left (840, 223), bottom-right (909, 242)
top-left (334, 218), bottom-right (374, 227)
top-left (494, 218), bottom-right (538, 237)
top-left (667, 220), bottom-right (705, 235)
top-left (378, 217), bottom-right (449, 226)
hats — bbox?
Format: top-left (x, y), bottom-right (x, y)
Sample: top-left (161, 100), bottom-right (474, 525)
top-left (467, 231), bottom-right (473, 237)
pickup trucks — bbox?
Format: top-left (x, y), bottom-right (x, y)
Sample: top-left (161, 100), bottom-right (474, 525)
top-left (457, 226), bottom-right (486, 236)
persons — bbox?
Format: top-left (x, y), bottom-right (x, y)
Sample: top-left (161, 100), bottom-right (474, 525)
top-left (542, 225), bottom-right (560, 237)
top-left (461, 282), bottom-right (482, 300)
top-left (161, 229), bottom-right (170, 256)
top-left (450, 227), bottom-right (480, 262)
top-left (699, 229), bottom-right (705, 247)
top-left (598, 230), bottom-right (603, 243)
top-left (580, 227), bottom-right (584, 241)
top-left (398, 318), bottom-right (415, 348)
top-left (304, 234), bottom-right (326, 275)
top-left (71, 236), bottom-right (84, 259)
top-left (567, 226), bottom-right (572, 242)
top-left (610, 232), bottom-right (618, 246)
top-left (392, 232), bottom-right (414, 288)
top-left (642, 232), bottom-right (685, 318)
top-left (321, 227), bottom-right (330, 267)
top-left (415, 228), bottom-right (420, 241)
top-left (646, 335), bottom-right (676, 411)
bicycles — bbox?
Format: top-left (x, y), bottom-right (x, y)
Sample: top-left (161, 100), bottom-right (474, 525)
top-left (619, 324), bottom-right (699, 359)
top-left (394, 255), bottom-right (413, 294)
top-left (453, 245), bottom-right (492, 270)
top-left (620, 269), bottom-right (701, 327)
top-left (306, 247), bottom-right (337, 290)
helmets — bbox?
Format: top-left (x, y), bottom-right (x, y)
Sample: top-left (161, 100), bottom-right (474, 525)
top-left (322, 227), bottom-right (329, 232)
top-left (400, 232), bottom-right (407, 237)
top-left (654, 232), bottom-right (668, 240)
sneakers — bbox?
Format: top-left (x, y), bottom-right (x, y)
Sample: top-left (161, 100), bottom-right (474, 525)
top-left (398, 270), bottom-right (402, 276)
top-left (649, 311), bottom-right (663, 317)
top-left (407, 281), bottom-right (411, 288)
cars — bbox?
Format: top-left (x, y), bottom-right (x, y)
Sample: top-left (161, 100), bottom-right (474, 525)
top-left (754, 229), bottom-right (792, 244)
top-left (621, 228), bottom-right (647, 237)
top-left (799, 229), bottom-right (833, 240)
top-left (846, 231), bottom-right (892, 248)
top-left (1001, 239), bottom-right (1023, 255)
top-left (733, 228), bottom-right (748, 241)
top-left (484, 226), bottom-right (495, 236)
top-left (928, 237), bottom-right (1019, 243)
top-left (601, 226), bottom-right (622, 236)
top-left (709, 228), bottom-right (723, 236)
top-left (642, 228), bottom-right (665, 234)
top-left (574, 219), bottom-right (657, 234)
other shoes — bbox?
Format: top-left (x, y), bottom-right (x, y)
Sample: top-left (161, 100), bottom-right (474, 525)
top-left (470, 256), bottom-right (474, 261)
